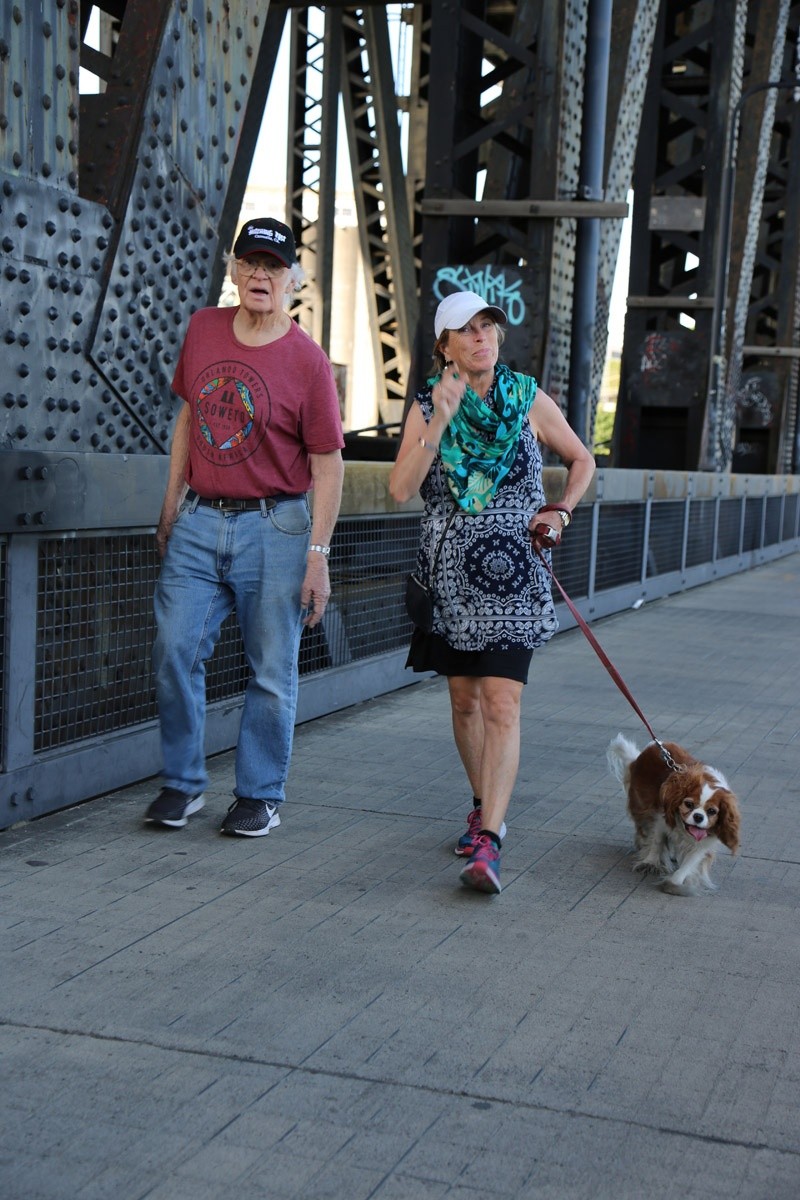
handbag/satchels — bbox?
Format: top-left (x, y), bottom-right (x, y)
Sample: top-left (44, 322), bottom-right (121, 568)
top-left (404, 572), bottom-right (434, 634)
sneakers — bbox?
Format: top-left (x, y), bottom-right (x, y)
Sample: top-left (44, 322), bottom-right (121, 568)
top-left (453, 805), bottom-right (508, 856)
top-left (143, 787), bottom-right (207, 829)
top-left (458, 835), bottom-right (503, 894)
top-left (218, 797), bottom-right (281, 837)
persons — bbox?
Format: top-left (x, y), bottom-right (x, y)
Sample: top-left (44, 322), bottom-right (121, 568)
top-left (386, 289), bottom-right (596, 896)
top-left (141, 216), bottom-right (345, 839)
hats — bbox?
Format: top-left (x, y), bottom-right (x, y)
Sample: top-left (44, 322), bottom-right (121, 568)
top-left (434, 291), bottom-right (508, 339)
top-left (233, 218), bottom-right (297, 269)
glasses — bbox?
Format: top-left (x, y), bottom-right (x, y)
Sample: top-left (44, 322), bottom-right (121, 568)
top-left (236, 256), bottom-right (285, 279)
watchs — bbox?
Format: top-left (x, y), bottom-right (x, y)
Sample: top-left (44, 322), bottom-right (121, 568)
top-left (305, 544), bottom-right (331, 560)
top-left (557, 509), bottom-right (571, 527)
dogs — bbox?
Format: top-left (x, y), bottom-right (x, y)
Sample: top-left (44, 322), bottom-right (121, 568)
top-left (602, 731), bottom-right (746, 897)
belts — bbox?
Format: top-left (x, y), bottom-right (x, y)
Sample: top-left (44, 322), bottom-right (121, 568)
top-left (184, 488), bottom-right (306, 511)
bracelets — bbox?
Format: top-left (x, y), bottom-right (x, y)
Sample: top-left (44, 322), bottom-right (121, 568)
top-left (418, 436), bottom-right (438, 453)
